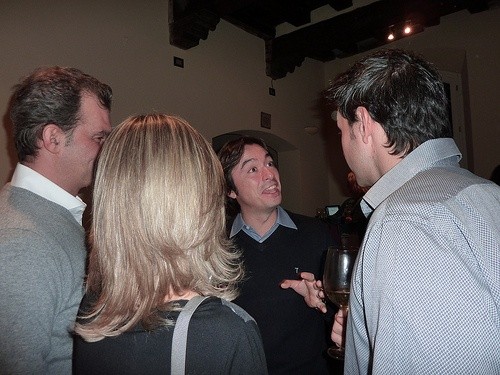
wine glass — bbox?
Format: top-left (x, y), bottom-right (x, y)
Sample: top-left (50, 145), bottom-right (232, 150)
top-left (323, 246), bottom-right (358, 359)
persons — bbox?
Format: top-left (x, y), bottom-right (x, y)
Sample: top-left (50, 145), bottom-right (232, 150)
top-left (216, 132), bottom-right (349, 375)
top-left (70, 113), bottom-right (269, 375)
top-left (324, 49), bottom-right (500, 375)
top-left (0, 65), bottom-right (113, 375)
